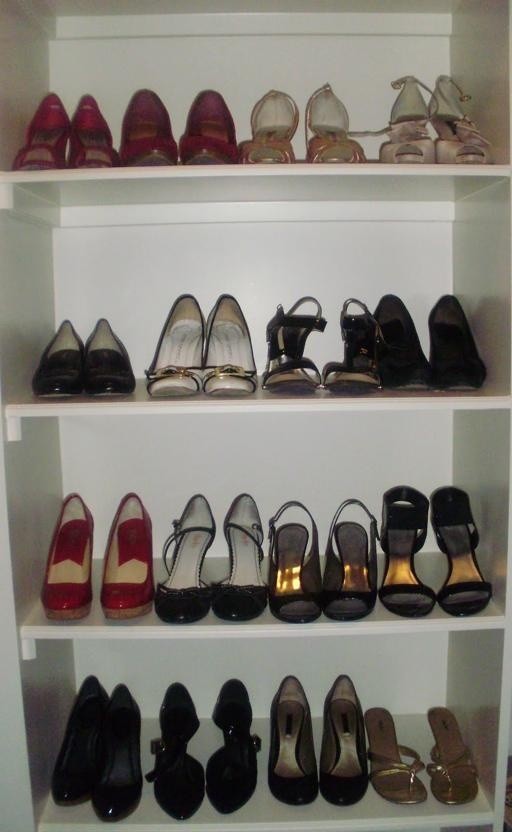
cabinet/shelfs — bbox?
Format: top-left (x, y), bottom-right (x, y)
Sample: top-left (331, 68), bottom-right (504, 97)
top-left (0, 0), bottom-right (511, 832)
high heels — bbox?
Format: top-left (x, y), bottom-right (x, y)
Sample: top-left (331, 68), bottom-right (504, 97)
top-left (119, 88), bottom-right (179, 167)
top-left (372, 293), bottom-right (429, 392)
top-left (261, 295), bottom-right (327, 395)
top-left (428, 292), bottom-right (487, 392)
top-left (238, 89), bottom-right (300, 165)
top-left (69, 93), bottom-right (115, 169)
top-left (206, 677), bottom-right (262, 816)
top-left (32, 318), bottom-right (84, 399)
top-left (425, 705), bottom-right (480, 806)
top-left (144, 292), bottom-right (207, 398)
top-left (378, 483), bottom-right (437, 619)
top-left (364, 706), bottom-right (428, 806)
top-left (267, 499), bottom-right (323, 624)
top-left (210, 492), bottom-right (269, 622)
top-left (203, 292), bottom-right (259, 397)
top-left (323, 497), bottom-right (379, 622)
top-left (85, 318), bottom-right (136, 398)
top-left (304, 81), bottom-right (367, 164)
top-left (144, 681), bottom-right (206, 820)
top-left (100, 491), bottom-right (155, 620)
top-left (154, 493), bottom-right (216, 624)
top-left (41, 491), bottom-right (95, 622)
top-left (320, 673), bottom-right (369, 807)
top-left (50, 673), bottom-right (111, 807)
top-left (321, 296), bottom-right (385, 395)
top-left (378, 75), bottom-right (436, 164)
top-left (427, 74), bottom-right (491, 164)
top-left (179, 89), bottom-right (239, 166)
top-left (266, 673), bottom-right (319, 807)
top-left (428, 484), bottom-right (494, 618)
top-left (12, 92), bottom-right (71, 172)
top-left (90, 682), bottom-right (144, 823)
